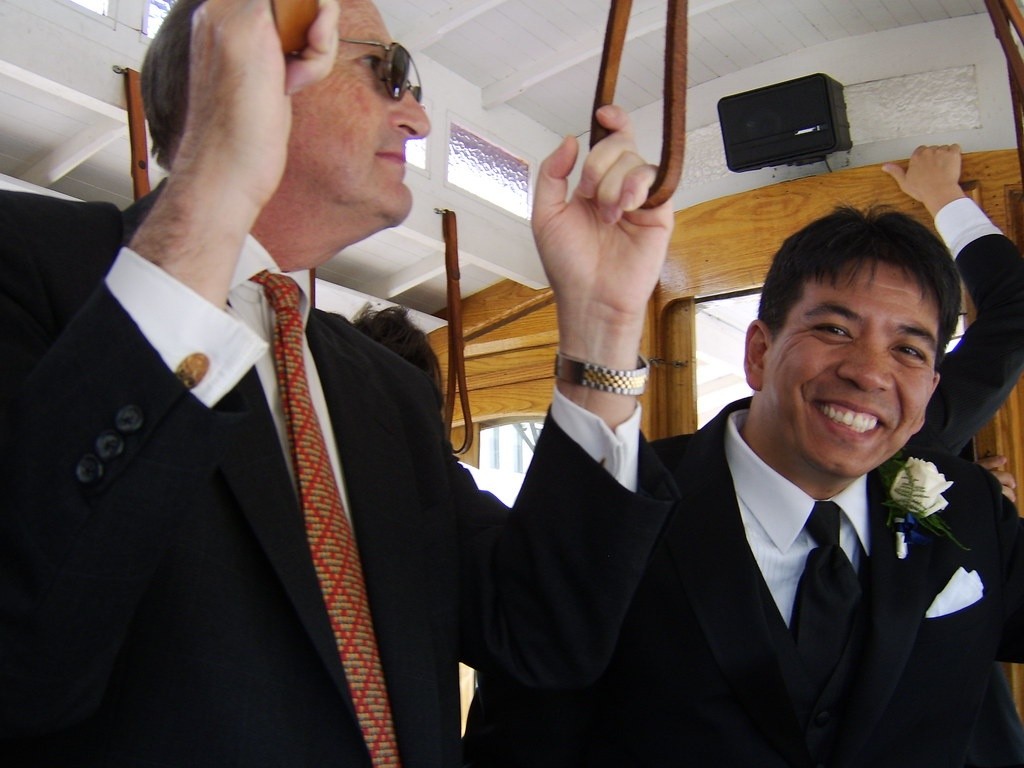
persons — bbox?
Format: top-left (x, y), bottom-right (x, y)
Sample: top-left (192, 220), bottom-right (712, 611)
top-left (460, 144), bottom-right (1024, 768)
top-left (0, 0), bottom-right (674, 768)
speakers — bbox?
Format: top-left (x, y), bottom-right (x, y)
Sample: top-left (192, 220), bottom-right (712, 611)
top-left (718, 73), bottom-right (851, 172)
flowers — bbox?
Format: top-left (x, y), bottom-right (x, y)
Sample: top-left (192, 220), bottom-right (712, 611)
top-left (874, 454), bottom-right (973, 558)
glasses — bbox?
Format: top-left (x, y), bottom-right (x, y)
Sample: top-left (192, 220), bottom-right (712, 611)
top-left (338, 39), bottom-right (425, 102)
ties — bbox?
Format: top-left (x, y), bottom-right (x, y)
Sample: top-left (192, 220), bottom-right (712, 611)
top-left (788, 501), bottom-right (864, 693)
top-left (249, 268), bottom-right (399, 768)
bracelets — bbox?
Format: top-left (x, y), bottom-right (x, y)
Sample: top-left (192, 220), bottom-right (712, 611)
top-left (554, 353), bottom-right (650, 395)
